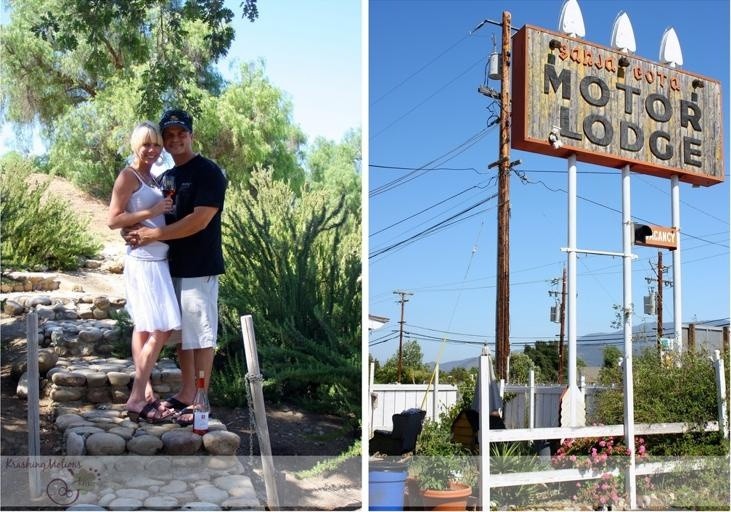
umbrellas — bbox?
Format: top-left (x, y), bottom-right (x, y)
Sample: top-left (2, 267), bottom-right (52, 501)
top-left (469, 341), bottom-right (502, 415)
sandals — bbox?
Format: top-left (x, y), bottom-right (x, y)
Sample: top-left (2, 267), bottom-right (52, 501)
top-left (151, 398), bottom-right (163, 411)
top-left (127, 402), bottom-right (176, 425)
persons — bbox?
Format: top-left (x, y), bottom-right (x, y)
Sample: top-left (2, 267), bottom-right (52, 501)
top-left (119, 108), bottom-right (226, 426)
top-left (105, 122), bottom-right (173, 424)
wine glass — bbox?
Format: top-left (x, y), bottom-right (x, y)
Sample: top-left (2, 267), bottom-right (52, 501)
top-left (159, 174), bottom-right (177, 213)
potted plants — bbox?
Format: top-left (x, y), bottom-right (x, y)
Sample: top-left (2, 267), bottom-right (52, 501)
top-left (409, 435), bottom-right (475, 511)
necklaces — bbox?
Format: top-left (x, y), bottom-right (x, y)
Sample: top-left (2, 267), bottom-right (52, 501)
top-left (132, 161), bottom-right (155, 188)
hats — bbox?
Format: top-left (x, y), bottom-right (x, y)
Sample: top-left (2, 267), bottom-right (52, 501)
top-left (159, 109), bottom-right (194, 130)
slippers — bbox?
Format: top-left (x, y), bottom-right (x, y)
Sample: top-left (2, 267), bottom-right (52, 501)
top-left (164, 396), bottom-right (188, 410)
top-left (172, 404), bottom-right (214, 427)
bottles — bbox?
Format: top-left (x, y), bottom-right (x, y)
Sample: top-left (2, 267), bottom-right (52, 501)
top-left (192, 379), bottom-right (212, 434)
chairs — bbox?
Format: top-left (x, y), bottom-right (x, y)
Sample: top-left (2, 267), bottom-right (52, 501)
top-left (369, 409), bottom-right (428, 457)
